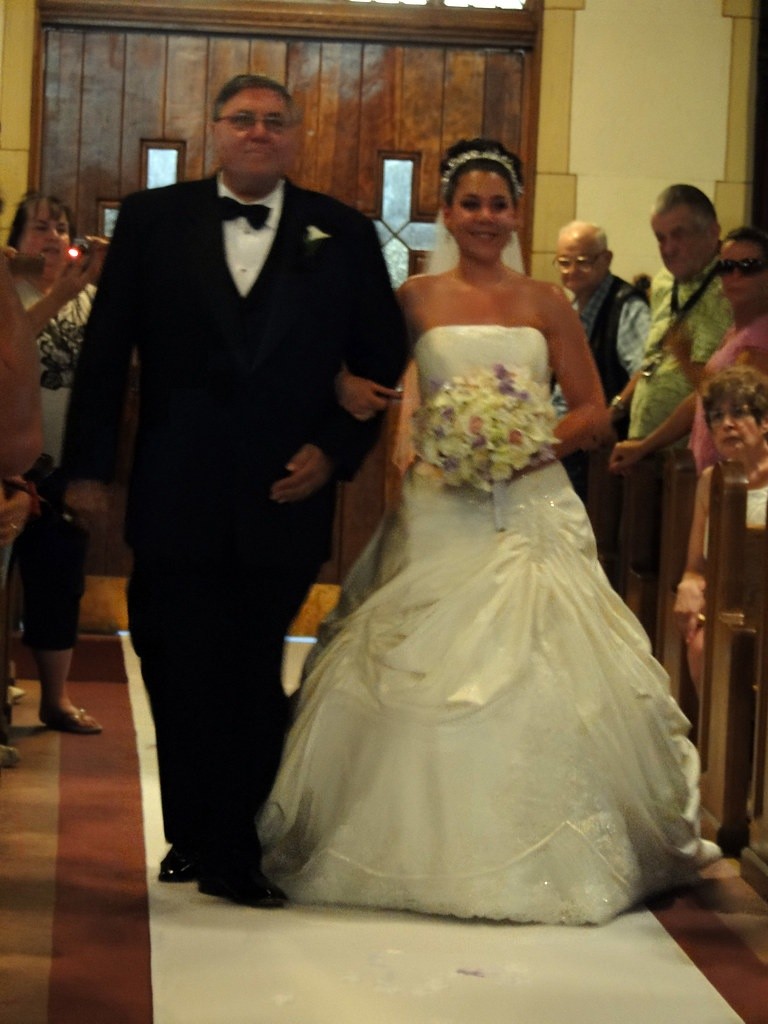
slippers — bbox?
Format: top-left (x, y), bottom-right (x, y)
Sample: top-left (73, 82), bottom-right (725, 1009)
top-left (44, 708), bottom-right (103, 733)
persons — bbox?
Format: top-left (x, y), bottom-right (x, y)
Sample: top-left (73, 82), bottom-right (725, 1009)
top-left (258, 137), bottom-right (704, 925)
top-left (8, 194), bottom-right (111, 733)
top-left (59, 75), bottom-right (411, 907)
top-left (0, 193), bottom-right (44, 770)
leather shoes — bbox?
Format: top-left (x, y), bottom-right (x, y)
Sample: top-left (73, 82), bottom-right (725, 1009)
top-left (158, 844), bottom-right (194, 882)
top-left (198, 872), bottom-right (289, 907)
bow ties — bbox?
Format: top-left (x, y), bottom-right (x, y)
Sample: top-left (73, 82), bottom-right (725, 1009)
top-left (219, 197), bottom-right (271, 231)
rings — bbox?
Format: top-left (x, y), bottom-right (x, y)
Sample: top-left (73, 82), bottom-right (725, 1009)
top-left (7, 522), bottom-right (20, 532)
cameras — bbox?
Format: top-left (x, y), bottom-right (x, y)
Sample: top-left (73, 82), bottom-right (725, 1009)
top-left (67, 238), bottom-right (89, 270)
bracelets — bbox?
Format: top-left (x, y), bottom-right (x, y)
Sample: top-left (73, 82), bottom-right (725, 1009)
top-left (610, 395), bottom-right (625, 409)
top-left (552, 183), bottom-right (768, 772)
top-left (675, 579), bottom-right (703, 591)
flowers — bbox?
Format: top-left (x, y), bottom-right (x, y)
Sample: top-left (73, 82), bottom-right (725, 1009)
top-left (418, 363), bottom-right (566, 493)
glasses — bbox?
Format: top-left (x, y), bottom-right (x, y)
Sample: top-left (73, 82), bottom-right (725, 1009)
top-left (708, 405), bottom-right (757, 423)
top-left (215, 114), bottom-right (289, 131)
top-left (552, 252), bottom-right (600, 273)
top-left (716, 258), bottom-right (766, 276)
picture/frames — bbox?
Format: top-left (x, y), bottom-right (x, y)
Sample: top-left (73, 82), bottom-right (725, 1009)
top-left (97, 201), bottom-right (122, 241)
